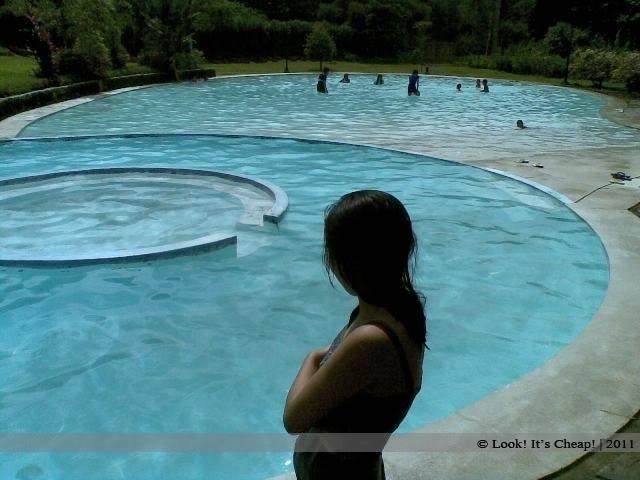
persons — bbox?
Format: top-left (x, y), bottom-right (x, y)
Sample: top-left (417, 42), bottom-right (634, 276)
top-left (407, 70), bottom-right (420, 96)
top-left (283, 189), bottom-right (427, 480)
top-left (373, 74), bottom-right (384, 85)
top-left (339, 73), bottom-right (350, 82)
top-left (323, 67), bottom-right (329, 92)
top-left (516, 120), bottom-right (527, 131)
top-left (476, 78), bottom-right (480, 87)
top-left (456, 83), bottom-right (462, 92)
top-left (480, 79), bottom-right (489, 92)
top-left (317, 74), bottom-right (326, 93)
